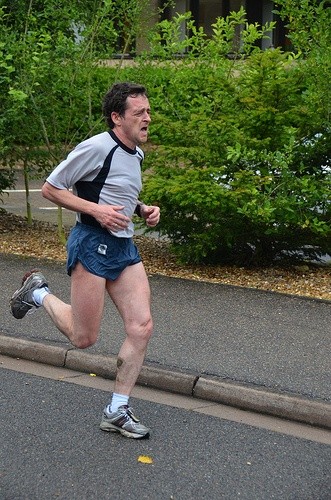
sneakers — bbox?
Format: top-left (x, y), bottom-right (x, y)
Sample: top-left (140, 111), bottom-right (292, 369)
top-left (100, 405), bottom-right (150, 439)
top-left (9, 268), bottom-right (48, 319)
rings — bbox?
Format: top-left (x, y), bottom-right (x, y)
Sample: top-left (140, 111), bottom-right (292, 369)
top-left (114, 224), bottom-right (117, 228)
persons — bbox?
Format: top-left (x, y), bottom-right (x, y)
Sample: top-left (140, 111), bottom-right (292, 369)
top-left (9, 82), bottom-right (160, 440)
